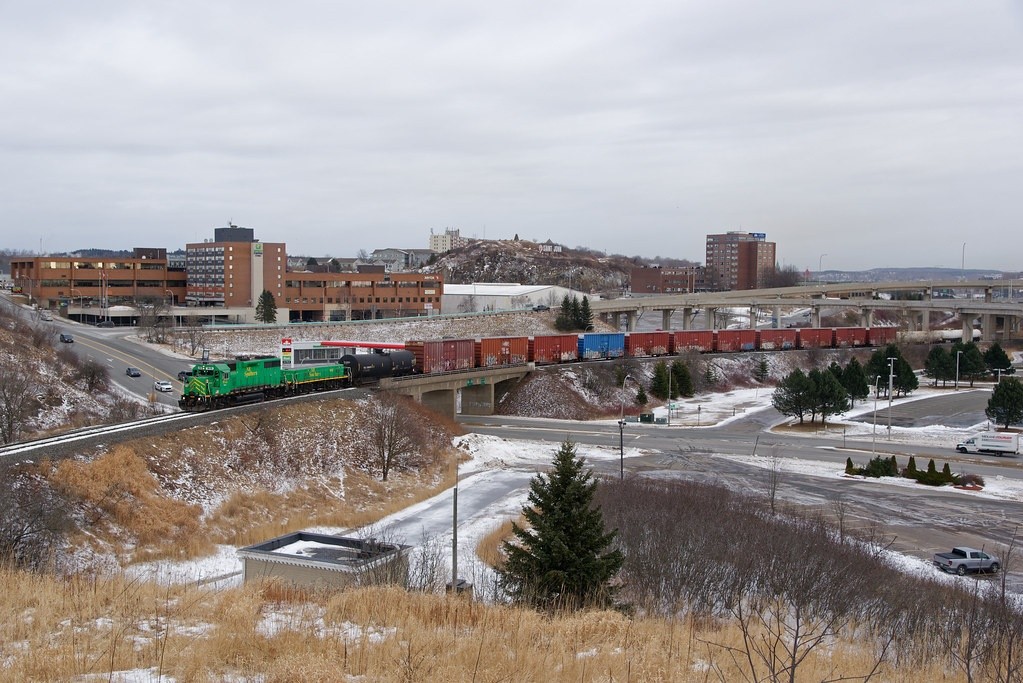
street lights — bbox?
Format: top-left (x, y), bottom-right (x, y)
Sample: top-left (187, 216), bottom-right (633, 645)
top-left (323, 286), bottom-right (329, 321)
top-left (22, 275), bottom-right (31, 308)
top-left (666, 365), bottom-right (671, 426)
top-left (873, 357), bottom-right (898, 459)
top-left (994, 368), bottom-right (1006, 384)
top-left (621, 375), bottom-right (630, 421)
top-left (618, 421), bottom-right (626, 481)
top-left (71, 289), bottom-right (82, 323)
top-left (166, 290), bottom-right (174, 325)
top-left (956, 351), bottom-right (963, 390)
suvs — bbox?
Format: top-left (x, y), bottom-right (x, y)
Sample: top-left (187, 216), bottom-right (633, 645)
top-left (97, 321), bottom-right (115, 328)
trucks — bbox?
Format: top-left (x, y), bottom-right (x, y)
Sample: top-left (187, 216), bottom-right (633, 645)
top-left (956, 433), bottom-right (1019, 457)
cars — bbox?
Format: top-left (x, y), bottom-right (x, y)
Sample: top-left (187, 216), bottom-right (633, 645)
top-left (154, 380), bottom-right (172, 392)
top-left (42, 313), bottom-right (53, 321)
top-left (61, 333), bottom-right (74, 343)
top-left (532, 304), bottom-right (550, 311)
top-left (126, 367), bottom-right (141, 377)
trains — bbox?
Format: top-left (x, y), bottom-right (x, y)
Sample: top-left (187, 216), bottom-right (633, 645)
top-left (178, 327), bottom-right (896, 413)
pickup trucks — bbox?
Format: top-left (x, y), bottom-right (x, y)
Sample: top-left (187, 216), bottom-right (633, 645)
top-left (178, 370), bottom-right (192, 381)
top-left (933, 546), bottom-right (1000, 576)
